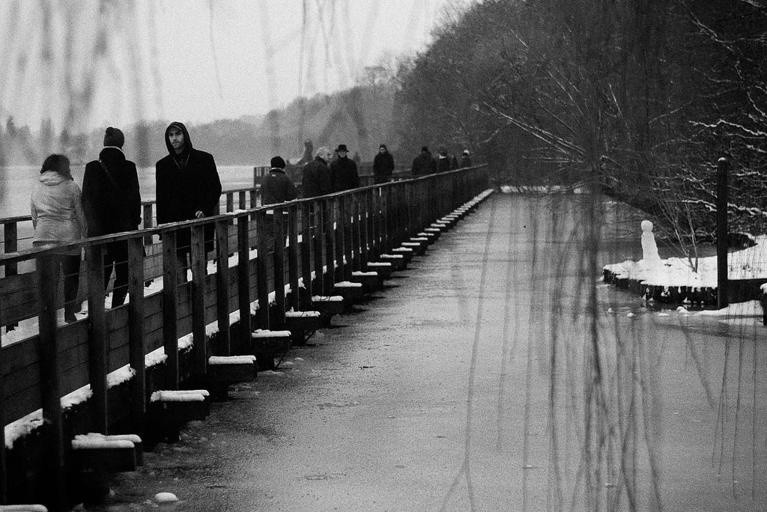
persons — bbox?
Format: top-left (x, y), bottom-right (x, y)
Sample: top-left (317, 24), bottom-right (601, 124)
top-left (302, 147), bottom-right (333, 233)
top-left (28, 153), bottom-right (87, 332)
top-left (412, 146), bottom-right (437, 178)
top-left (81, 126), bottom-right (143, 311)
top-left (257, 155), bottom-right (299, 248)
top-left (372, 144), bottom-right (393, 200)
top-left (330, 143), bottom-right (360, 219)
top-left (155, 121), bottom-right (221, 281)
top-left (436, 149), bottom-right (471, 173)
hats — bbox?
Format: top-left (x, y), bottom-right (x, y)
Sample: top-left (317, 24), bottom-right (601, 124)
top-left (270, 155), bottom-right (286, 170)
top-left (335, 144), bottom-right (349, 153)
top-left (103, 126), bottom-right (125, 148)
